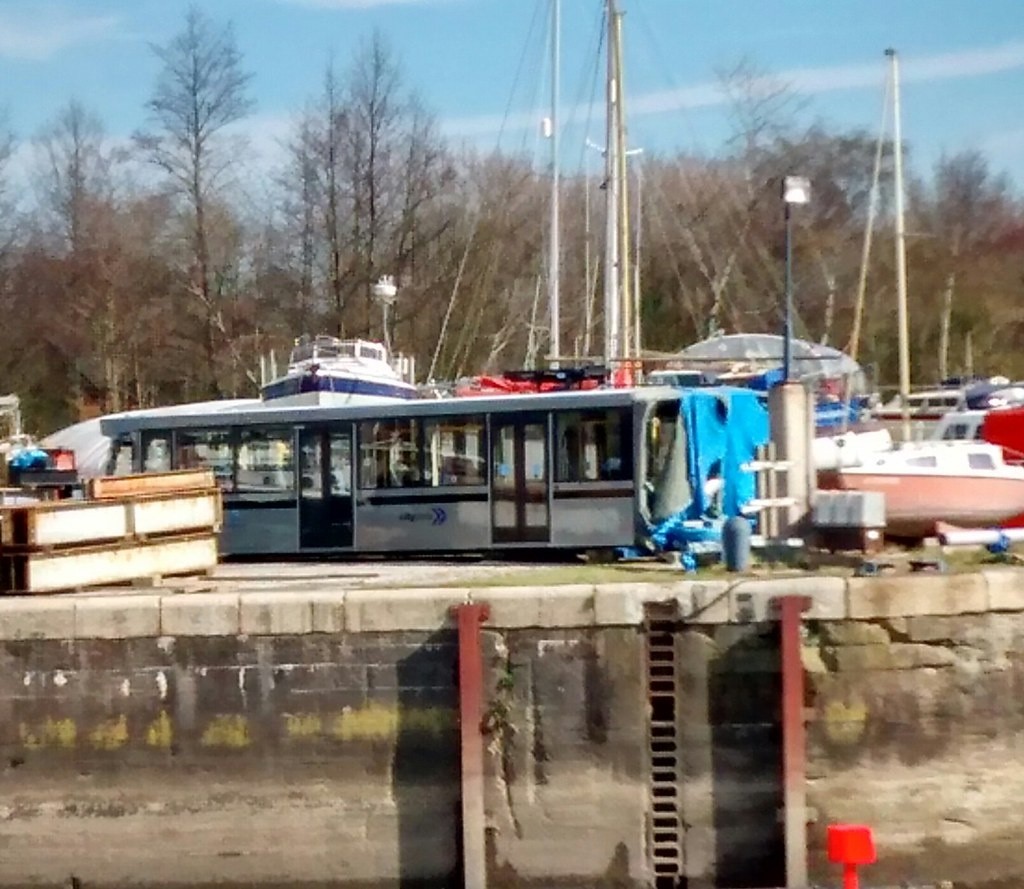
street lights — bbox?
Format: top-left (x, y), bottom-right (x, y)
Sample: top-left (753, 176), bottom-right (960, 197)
top-left (779, 175), bottom-right (810, 381)
top-left (372, 273), bottom-right (398, 351)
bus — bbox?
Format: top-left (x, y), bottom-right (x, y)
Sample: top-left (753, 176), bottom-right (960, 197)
top-left (101, 385), bottom-right (718, 557)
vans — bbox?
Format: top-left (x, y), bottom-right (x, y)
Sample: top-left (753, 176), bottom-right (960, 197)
top-left (873, 390), bottom-right (967, 419)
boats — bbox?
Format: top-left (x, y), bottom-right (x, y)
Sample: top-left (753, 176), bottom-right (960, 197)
top-left (258, 336), bottom-right (422, 406)
top-left (818, 437), bottom-right (1024, 538)
top-left (838, 48), bottom-right (1024, 464)
